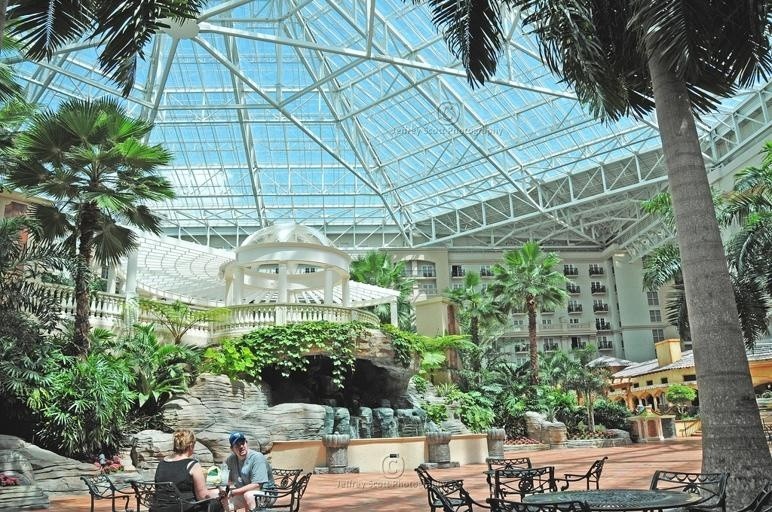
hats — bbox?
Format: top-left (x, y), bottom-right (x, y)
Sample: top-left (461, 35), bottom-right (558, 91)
top-left (229, 432), bottom-right (248, 447)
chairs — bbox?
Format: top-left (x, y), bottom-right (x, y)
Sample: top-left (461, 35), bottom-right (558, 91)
top-left (649, 464), bottom-right (731, 510)
top-left (80, 464), bottom-right (314, 511)
top-left (414, 453), bottom-right (612, 512)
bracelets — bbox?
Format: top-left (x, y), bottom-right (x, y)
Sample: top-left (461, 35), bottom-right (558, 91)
top-left (230, 490), bottom-right (234, 498)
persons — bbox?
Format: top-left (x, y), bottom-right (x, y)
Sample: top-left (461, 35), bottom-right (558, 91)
top-left (95, 450), bottom-right (121, 468)
top-left (219, 431), bottom-right (278, 512)
top-left (148, 430), bottom-right (225, 512)
top-left (631, 404), bottom-right (643, 416)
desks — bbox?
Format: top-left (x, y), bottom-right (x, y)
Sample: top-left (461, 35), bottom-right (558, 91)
top-left (521, 487), bottom-right (703, 512)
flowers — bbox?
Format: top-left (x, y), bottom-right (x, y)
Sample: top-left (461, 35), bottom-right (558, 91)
top-left (92, 454), bottom-right (125, 475)
top-left (0, 469), bottom-right (20, 487)
